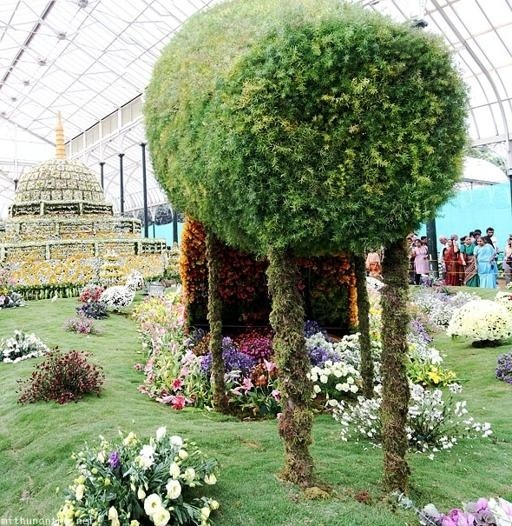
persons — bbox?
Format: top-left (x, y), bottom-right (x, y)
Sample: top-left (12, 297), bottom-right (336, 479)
top-left (365, 228), bottom-right (511, 287)
